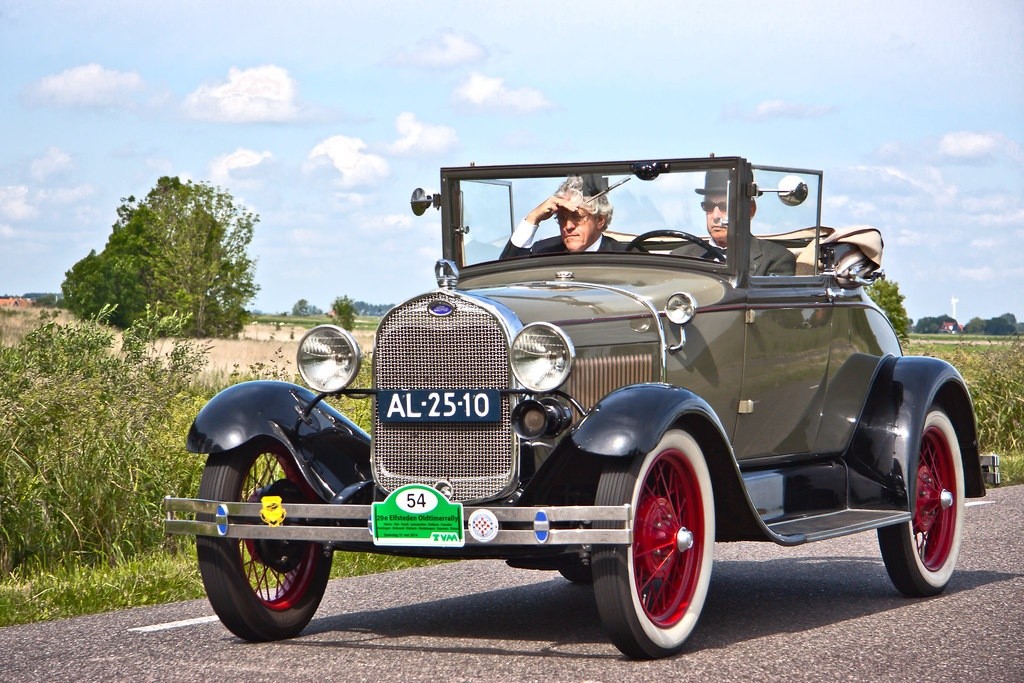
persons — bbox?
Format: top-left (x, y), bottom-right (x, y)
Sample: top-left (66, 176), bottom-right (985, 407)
top-left (671, 167), bottom-right (796, 278)
top-left (500, 174), bottom-right (640, 261)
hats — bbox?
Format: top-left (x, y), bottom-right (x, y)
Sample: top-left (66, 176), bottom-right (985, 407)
top-left (695, 168), bottom-right (763, 196)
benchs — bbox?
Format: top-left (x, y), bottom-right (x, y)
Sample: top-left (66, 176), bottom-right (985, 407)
top-left (604, 228), bottom-right (827, 278)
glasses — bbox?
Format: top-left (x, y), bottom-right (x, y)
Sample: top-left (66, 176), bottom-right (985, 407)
top-left (701, 201), bottom-right (727, 212)
top-left (554, 212), bottom-right (590, 225)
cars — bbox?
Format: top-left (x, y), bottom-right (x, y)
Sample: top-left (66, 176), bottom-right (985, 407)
top-left (164, 154), bottom-right (1002, 660)
